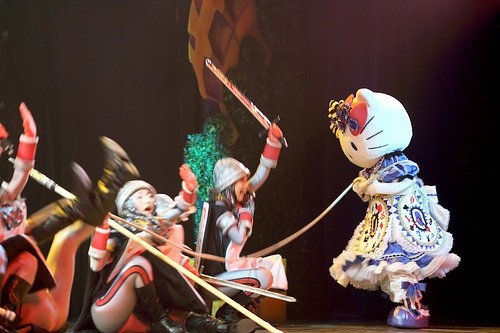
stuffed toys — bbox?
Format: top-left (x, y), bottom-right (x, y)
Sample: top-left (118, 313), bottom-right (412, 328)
top-left (329, 88), bottom-right (461, 329)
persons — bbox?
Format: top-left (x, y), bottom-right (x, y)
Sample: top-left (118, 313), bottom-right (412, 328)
top-left (151, 193), bottom-right (184, 257)
top-left (195, 126), bottom-right (289, 321)
top-left (0, 101), bottom-right (140, 333)
top-left (91, 181), bottom-right (255, 333)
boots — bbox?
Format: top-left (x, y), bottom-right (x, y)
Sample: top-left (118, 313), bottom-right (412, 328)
top-left (185, 312), bottom-right (257, 332)
top-left (0, 275), bottom-right (32, 333)
top-left (135, 281), bottom-right (185, 333)
top-left (24, 162), bottom-right (105, 243)
top-left (82, 134), bottom-right (140, 225)
top-left (236, 300), bottom-right (259, 319)
top-left (215, 290), bottom-right (254, 320)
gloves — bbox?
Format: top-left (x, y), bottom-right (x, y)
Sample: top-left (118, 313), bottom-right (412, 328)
top-left (268, 123), bottom-right (282, 143)
top-left (98, 214), bottom-right (109, 229)
top-left (236, 203), bottom-right (243, 209)
top-left (179, 162), bottom-right (198, 191)
top-left (18, 101), bottom-right (37, 136)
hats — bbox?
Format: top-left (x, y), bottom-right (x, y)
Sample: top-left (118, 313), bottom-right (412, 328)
top-left (153, 192), bottom-right (176, 214)
top-left (212, 156), bottom-right (251, 192)
top-left (116, 179), bottom-right (156, 219)
